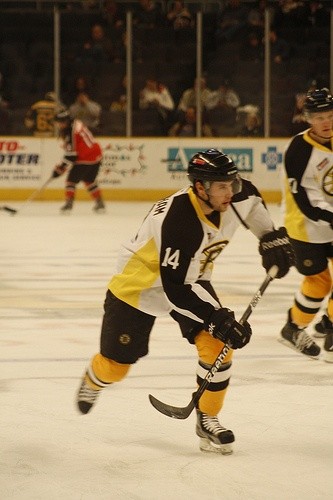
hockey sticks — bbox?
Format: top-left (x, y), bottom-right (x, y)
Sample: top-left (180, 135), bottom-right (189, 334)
top-left (3, 176), bottom-right (54, 213)
top-left (146, 265), bottom-right (279, 419)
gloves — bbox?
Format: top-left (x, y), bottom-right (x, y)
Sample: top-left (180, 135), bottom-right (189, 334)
top-left (259, 227), bottom-right (298, 279)
top-left (207, 307), bottom-right (253, 350)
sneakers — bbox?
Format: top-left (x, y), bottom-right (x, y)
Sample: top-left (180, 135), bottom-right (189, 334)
top-left (92, 201), bottom-right (106, 216)
top-left (59, 201), bottom-right (74, 214)
top-left (324, 330), bottom-right (333, 352)
top-left (276, 306), bottom-right (322, 361)
top-left (75, 374), bottom-right (100, 416)
top-left (313, 314), bottom-right (333, 338)
top-left (195, 411), bottom-right (236, 456)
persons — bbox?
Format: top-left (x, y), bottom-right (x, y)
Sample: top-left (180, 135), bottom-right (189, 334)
top-left (74, 147), bottom-right (298, 456)
top-left (138, 69), bottom-right (175, 120)
top-left (26, 0), bottom-right (330, 138)
top-left (52, 108), bottom-right (108, 212)
top-left (283, 85), bottom-right (333, 361)
top-left (167, 105), bottom-right (214, 137)
top-left (26, 89), bottom-right (69, 137)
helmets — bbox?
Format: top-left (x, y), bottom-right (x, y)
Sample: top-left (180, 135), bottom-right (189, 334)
top-left (302, 86), bottom-right (333, 113)
top-left (55, 109), bottom-right (72, 122)
top-left (186, 148), bottom-right (237, 185)
